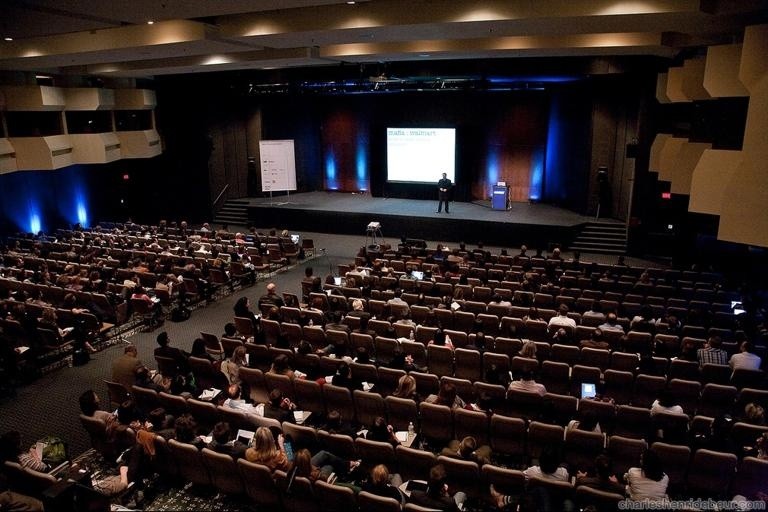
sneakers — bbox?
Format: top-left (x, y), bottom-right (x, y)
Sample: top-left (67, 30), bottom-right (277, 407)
top-left (348, 459), bottom-right (362, 474)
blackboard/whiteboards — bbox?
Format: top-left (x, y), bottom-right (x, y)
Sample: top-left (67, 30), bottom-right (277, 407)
top-left (259, 139), bottom-right (297, 191)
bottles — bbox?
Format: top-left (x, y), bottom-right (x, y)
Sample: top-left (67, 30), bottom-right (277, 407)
top-left (309, 319), bottom-right (313, 327)
top-left (410, 330), bottom-right (414, 340)
top-left (408, 422), bottom-right (414, 438)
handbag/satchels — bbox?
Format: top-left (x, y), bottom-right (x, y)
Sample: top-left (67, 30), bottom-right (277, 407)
top-left (283, 442), bottom-right (294, 462)
top-left (171, 305), bottom-right (192, 321)
top-left (170, 369), bottom-right (197, 394)
top-left (72, 347), bottom-right (91, 367)
top-left (31, 436), bottom-right (66, 463)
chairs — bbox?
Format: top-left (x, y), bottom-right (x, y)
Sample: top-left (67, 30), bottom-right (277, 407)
top-left (0, 221), bottom-right (768, 512)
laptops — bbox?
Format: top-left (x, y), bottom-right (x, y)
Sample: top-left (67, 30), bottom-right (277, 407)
top-left (581, 382), bottom-right (596, 398)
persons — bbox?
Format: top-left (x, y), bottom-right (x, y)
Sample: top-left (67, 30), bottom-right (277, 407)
top-left (435, 173), bottom-right (451, 213)
top-left (1, 220), bottom-right (766, 512)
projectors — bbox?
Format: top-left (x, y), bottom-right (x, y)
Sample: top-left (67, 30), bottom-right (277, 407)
top-left (368, 221), bottom-right (380, 229)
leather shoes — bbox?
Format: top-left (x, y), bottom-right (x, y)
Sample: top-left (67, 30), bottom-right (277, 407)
top-left (490, 483), bottom-right (504, 509)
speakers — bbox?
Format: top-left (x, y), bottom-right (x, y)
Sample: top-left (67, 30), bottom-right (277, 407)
top-left (407, 237), bottom-right (426, 249)
top-left (597, 166), bottom-right (609, 203)
top-left (248, 155), bottom-right (256, 192)
